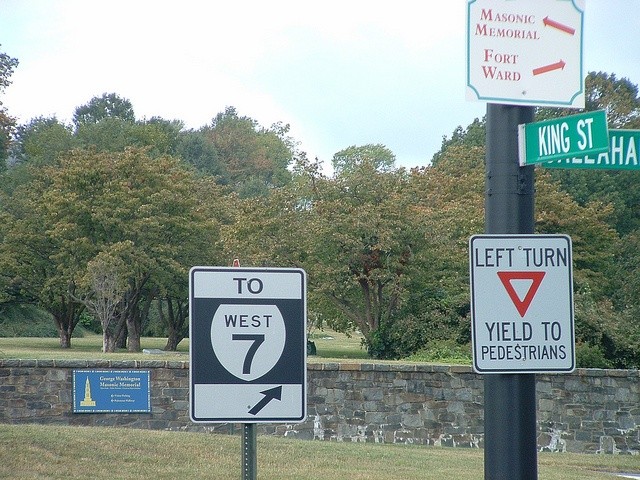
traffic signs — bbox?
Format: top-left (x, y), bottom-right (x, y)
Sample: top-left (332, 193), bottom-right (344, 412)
top-left (519, 110), bottom-right (611, 166)
top-left (189, 264), bottom-right (308, 426)
top-left (469, 234), bottom-right (576, 375)
top-left (540, 129), bottom-right (639, 169)
top-left (466, 0), bottom-right (585, 111)
